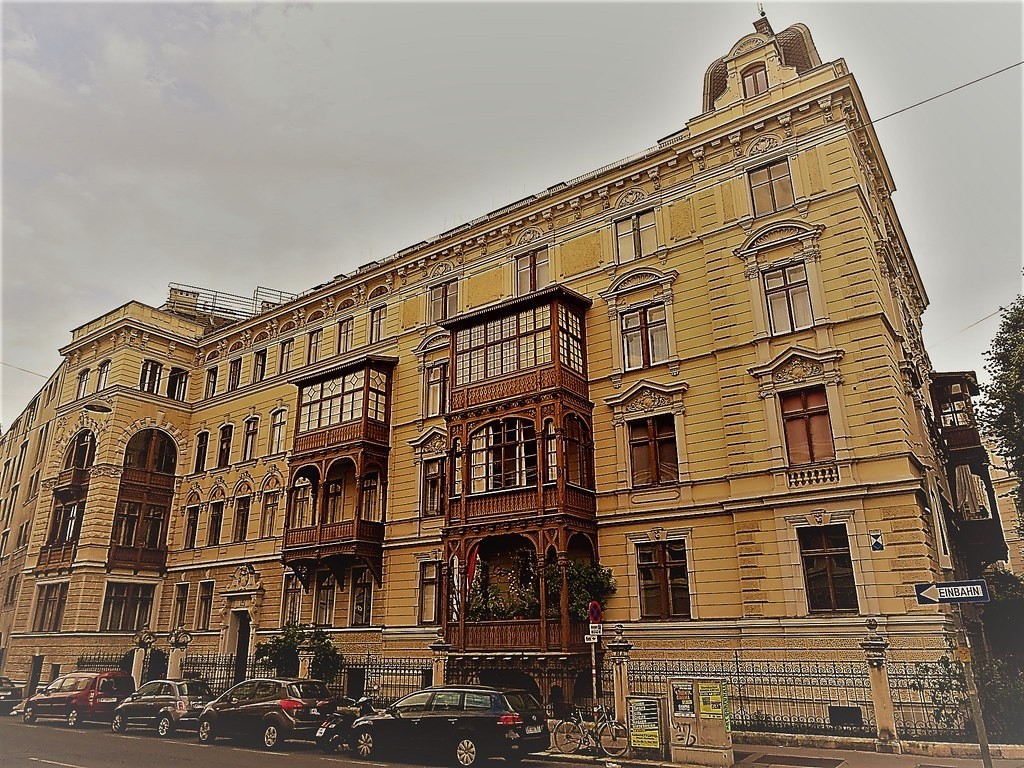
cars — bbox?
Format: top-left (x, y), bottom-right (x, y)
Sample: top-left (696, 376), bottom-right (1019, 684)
top-left (23, 672), bottom-right (137, 730)
top-left (0, 675), bottom-right (23, 715)
top-left (199, 677), bottom-right (339, 751)
top-left (110, 677), bottom-right (214, 740)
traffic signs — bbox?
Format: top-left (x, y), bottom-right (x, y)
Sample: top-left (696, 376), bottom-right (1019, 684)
top-left (915, 581), bottom-right (990, 607)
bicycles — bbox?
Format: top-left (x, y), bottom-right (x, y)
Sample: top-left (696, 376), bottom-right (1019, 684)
top-left (553, 706), bottom-right (629, 759)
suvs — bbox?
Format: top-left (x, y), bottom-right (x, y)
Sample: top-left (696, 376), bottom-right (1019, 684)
top-left (346, 683), bottom-right (550, 768)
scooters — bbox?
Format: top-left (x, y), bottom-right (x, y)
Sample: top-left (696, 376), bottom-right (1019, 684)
top-left (317, 685), bottom-right (385, 755)
